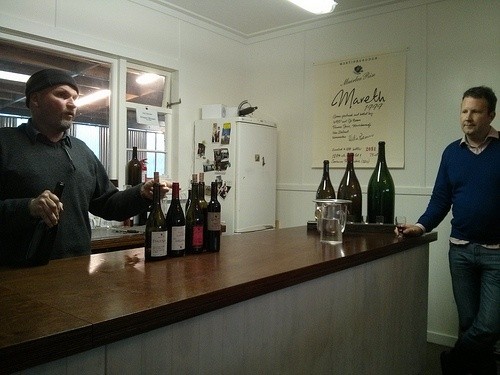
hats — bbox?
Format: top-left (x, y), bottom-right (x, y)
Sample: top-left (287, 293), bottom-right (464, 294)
top-left (24, 68), bottom-right (79, 108)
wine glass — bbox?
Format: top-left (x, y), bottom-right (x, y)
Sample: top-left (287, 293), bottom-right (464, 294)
top-left (395, 216), bottom-right (407, 238)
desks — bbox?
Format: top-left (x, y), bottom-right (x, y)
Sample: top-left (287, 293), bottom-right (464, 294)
top-left (90, 227), bottom-right (145, 254)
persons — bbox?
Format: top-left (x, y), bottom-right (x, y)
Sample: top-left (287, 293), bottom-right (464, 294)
top-left (394, 85), bottom-right (500, 375)
top-left (0, 68), bottom-right (182, 272)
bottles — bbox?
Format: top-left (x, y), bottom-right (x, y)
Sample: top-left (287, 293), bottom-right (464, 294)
top-left (127, 146), bottom-right (143, 186)
top-left (144, 172), bottom-right (168, 262)
top-left (185, 181), bottom-right (207, 255)
top-left (239, 106), bottom-right (258, 116)
top-left (207, 181), bottom-right (222, 253)
top-left (166, 182), bottom-right (186, 257)
top-left (22, 182), bottom-right (65, 265)
top-left (314, 159), bottom-right (335, 222)
top-left (366, 140), bottom-right (395, 225)
top-left (335, 152), bottom-right (362, 223)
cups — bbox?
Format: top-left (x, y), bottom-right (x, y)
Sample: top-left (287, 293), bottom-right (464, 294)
top-left (88, 215), bottom-right (113, 230)
top-left (319, 202), bottom-right (347, 242)
top-left (375, 215), bottom-right (384, 225)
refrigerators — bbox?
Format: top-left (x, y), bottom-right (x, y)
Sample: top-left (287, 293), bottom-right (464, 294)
top-left (194, 118), bottom-right (278, 236)
